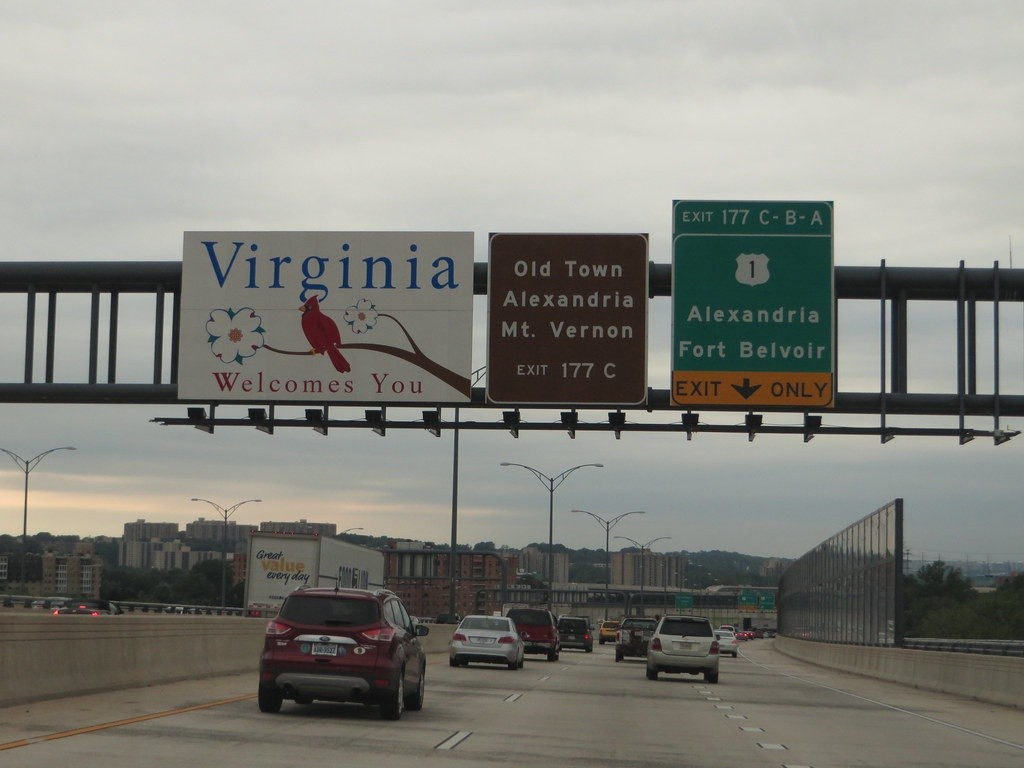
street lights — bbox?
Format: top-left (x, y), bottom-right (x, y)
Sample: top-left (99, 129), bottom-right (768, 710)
top-left (500, 461), bottom-right (605, 612)
top-left (191, 497), bottom-right (262, 616)
top-left (614, 535), bottom-right (672, 619)
top-left (570, 508), bottom-right (646, 622)
top-left (0, 446), bottom-right (77, 595)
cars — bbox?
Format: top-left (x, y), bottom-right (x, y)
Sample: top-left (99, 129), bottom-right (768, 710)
top-left (448, 614), bottom-right (526, 670)
top-left (713, 624), bottom-right (776, 658)
top-left (48, 598), bottom-right (124, 616)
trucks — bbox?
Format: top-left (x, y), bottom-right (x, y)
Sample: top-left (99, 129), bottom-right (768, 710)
top-left (240, 529), bottom-right (388, 619)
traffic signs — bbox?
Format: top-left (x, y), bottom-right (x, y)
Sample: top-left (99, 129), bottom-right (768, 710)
top-left (668, 197), bottom-right (837, 411)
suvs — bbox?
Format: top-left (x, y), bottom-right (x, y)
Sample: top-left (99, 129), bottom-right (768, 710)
top-left (556, 613), bottom-right (596, 653)
top-left (644, 614), bottom-right (721, 684)
top-left (615, 618), bottom-right (659, 663)
top-left (256, 585), bottom-right (430, 722)
top-left (499, 607), bottom-right (561, 662)
top-left (598, 620), bottom-right (620, 645)
